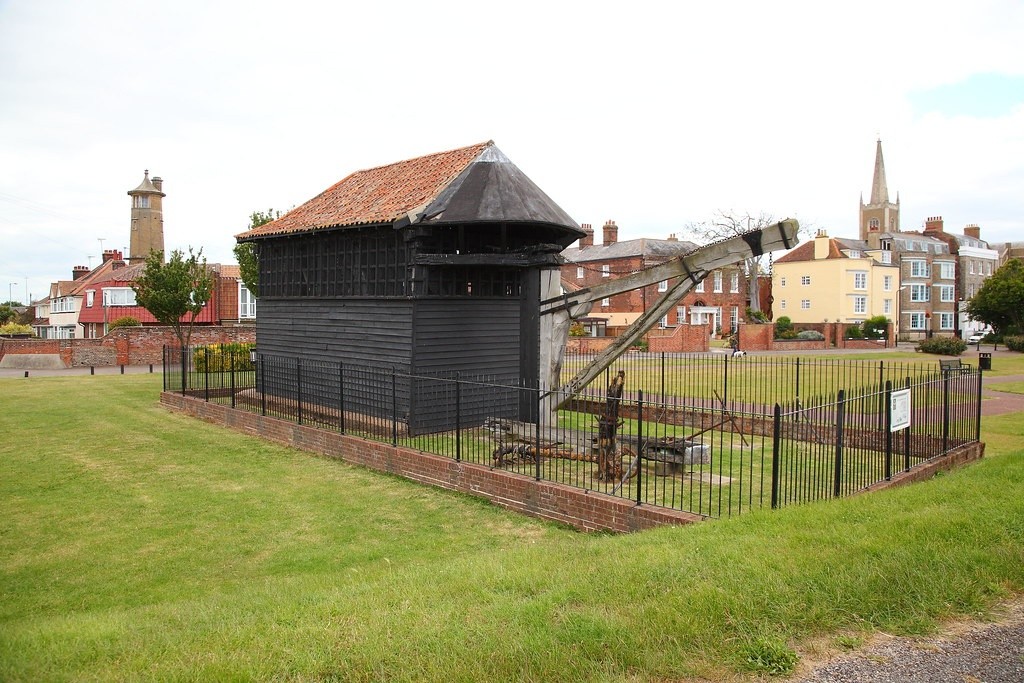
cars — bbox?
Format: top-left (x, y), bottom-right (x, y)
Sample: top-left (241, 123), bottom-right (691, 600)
top-left (968, 331), bottom-right (990, 345)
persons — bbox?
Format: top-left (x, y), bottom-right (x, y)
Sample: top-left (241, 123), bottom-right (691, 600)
top-left (731, 336), bottom-right (738, 357)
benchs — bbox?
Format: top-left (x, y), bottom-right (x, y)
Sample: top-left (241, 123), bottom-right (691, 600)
top-left (939, 359), bottom-right (970, 375)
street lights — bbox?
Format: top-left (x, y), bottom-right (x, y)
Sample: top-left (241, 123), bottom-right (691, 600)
top-left (896, 286), bottom-right (907, 347)
top-left (955, 297), bottom-right (962, 331)
top-left (10, 282), bottom-right (18, 309)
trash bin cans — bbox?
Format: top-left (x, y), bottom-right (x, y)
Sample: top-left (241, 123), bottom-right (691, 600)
top-left (979, 352), bottom-right (992, 370)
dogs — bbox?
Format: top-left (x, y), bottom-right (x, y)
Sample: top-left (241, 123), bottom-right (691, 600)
top-left (733, 350), bottom-right (747, 357)
